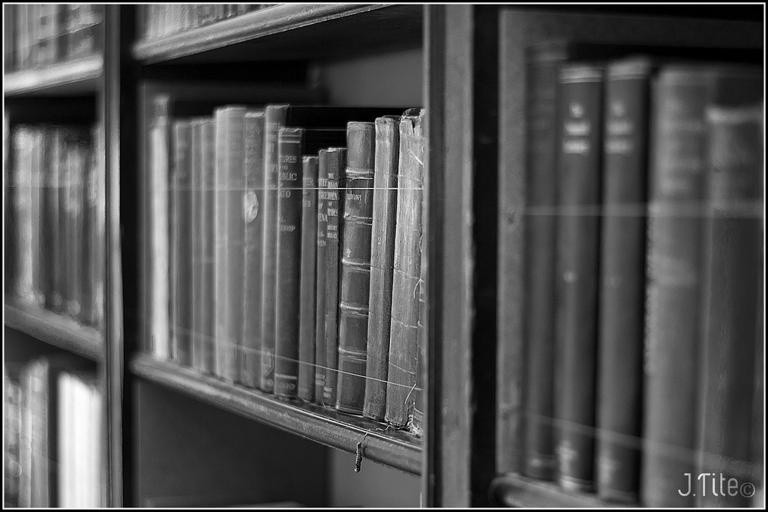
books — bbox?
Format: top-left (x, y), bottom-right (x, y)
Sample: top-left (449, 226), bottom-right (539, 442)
top-left (136, 4), bottom-right (266, 43)
top-left (132, 77), bottom-right (429, 442)
top-left (12, 5), bottom-right (103, 75)
top-left (9, 120), bottom-right (103, 333)
top-left (3, 356), bottom-right (107, 511)
top-left (513, 51), bottom-right (764, 509)
top-left (131, 378), bottom-right (329, 508)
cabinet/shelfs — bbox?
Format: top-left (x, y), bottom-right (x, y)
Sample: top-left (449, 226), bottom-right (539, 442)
top-left (4, 3), bottom-right (766, 509)
